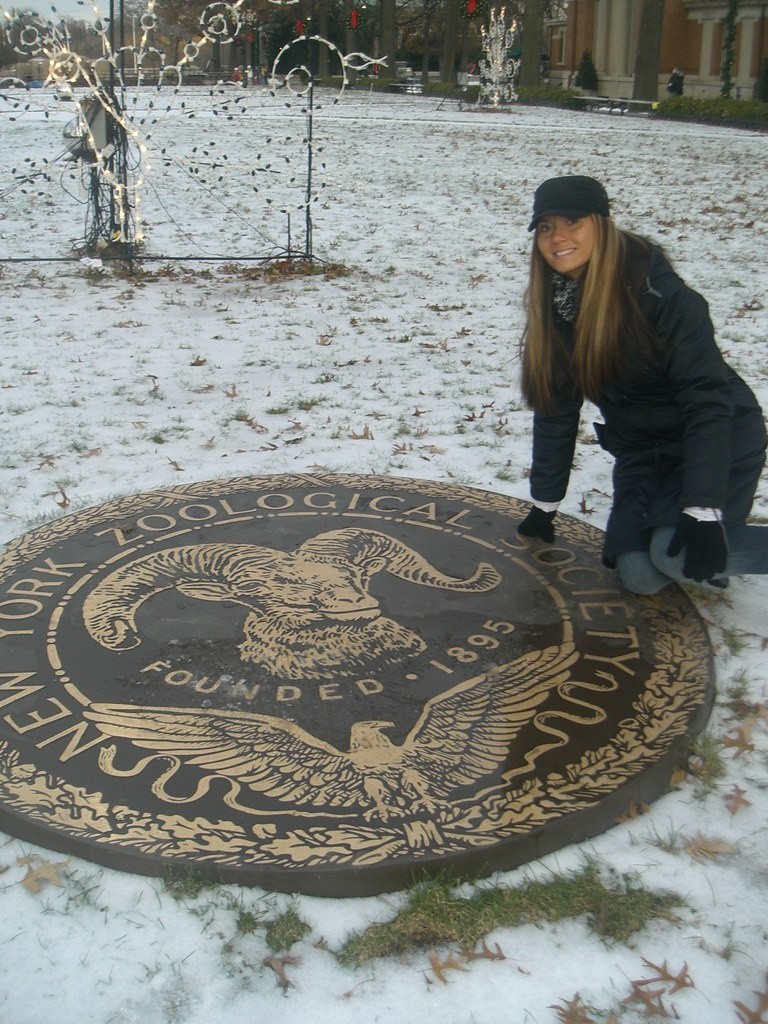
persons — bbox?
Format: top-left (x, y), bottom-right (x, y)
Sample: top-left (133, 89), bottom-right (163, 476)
top-left (232, 64), bottom-right (269, 86)
top-left (517, 173), bottom-right (768, 594)
top-left (666, 66), bottom-right (683, 97)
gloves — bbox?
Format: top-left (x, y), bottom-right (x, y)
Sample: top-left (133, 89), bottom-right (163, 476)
top-left (666, 510), bottom-right (728, 581)
top-left (517, 507), bottom-right (556, 546)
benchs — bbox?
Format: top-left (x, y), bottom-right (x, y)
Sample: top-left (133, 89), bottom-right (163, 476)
top-left (573, 95), bottom-right (661, 116)
top-left (389, 83), bottom-right (423, 94)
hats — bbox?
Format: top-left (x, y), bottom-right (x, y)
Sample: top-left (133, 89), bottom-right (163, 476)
top-left (527, 175), bottom-right (609, 231)
